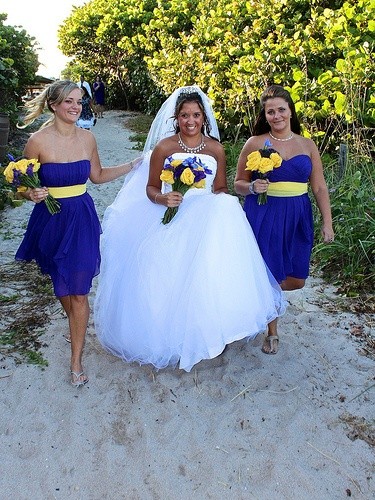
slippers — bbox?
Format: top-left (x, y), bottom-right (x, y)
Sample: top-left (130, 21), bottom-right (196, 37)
top-left (70, 369), bottom-right (88, 386)
top-left (262, 335), bottom-right (279, 354)
top-left (65, 330), bottom-right (72, 342)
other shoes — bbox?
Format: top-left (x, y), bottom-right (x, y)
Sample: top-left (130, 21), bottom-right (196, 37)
top-left (98, 116), bottom-right (104, 118)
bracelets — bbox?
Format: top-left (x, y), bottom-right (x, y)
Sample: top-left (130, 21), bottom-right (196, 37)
top-left (154, 193), bottom-right (161, 205)
top-left (130, 161), bottom-right (134, 169)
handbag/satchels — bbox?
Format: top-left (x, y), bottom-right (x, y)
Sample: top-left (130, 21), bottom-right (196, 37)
top-left (93, 119), bottom-right (97, 126)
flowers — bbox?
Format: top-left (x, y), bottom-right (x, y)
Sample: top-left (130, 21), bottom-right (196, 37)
top-left (159, 156), bottom-right (213, 224)
top-left (3, 158), bottom-right (61, 216)
top-left (244, 138), bottom-right (283, 206)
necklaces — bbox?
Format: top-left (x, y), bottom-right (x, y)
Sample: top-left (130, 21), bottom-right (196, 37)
top-left (178, 132), bottom-right (207, 154)
top-left (269, 130), bottom-right (294, 141)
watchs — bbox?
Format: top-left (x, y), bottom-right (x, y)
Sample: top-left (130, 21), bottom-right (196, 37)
top-left (248, 181), bottom-right (258, 195)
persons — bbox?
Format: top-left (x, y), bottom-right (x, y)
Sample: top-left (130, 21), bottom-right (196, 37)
top-left (92, 76), bottom-right (105, 118)
top-left (13, 79), bottom-right (142, 387)
top-left (75, 87), bottom-right (97, 131)
top-left (75, 77), bottom-right (93, 100)
top-left (233, 86), bottom-right (335, 355)
top-left (88, 86), bottom-right (289, 372)
